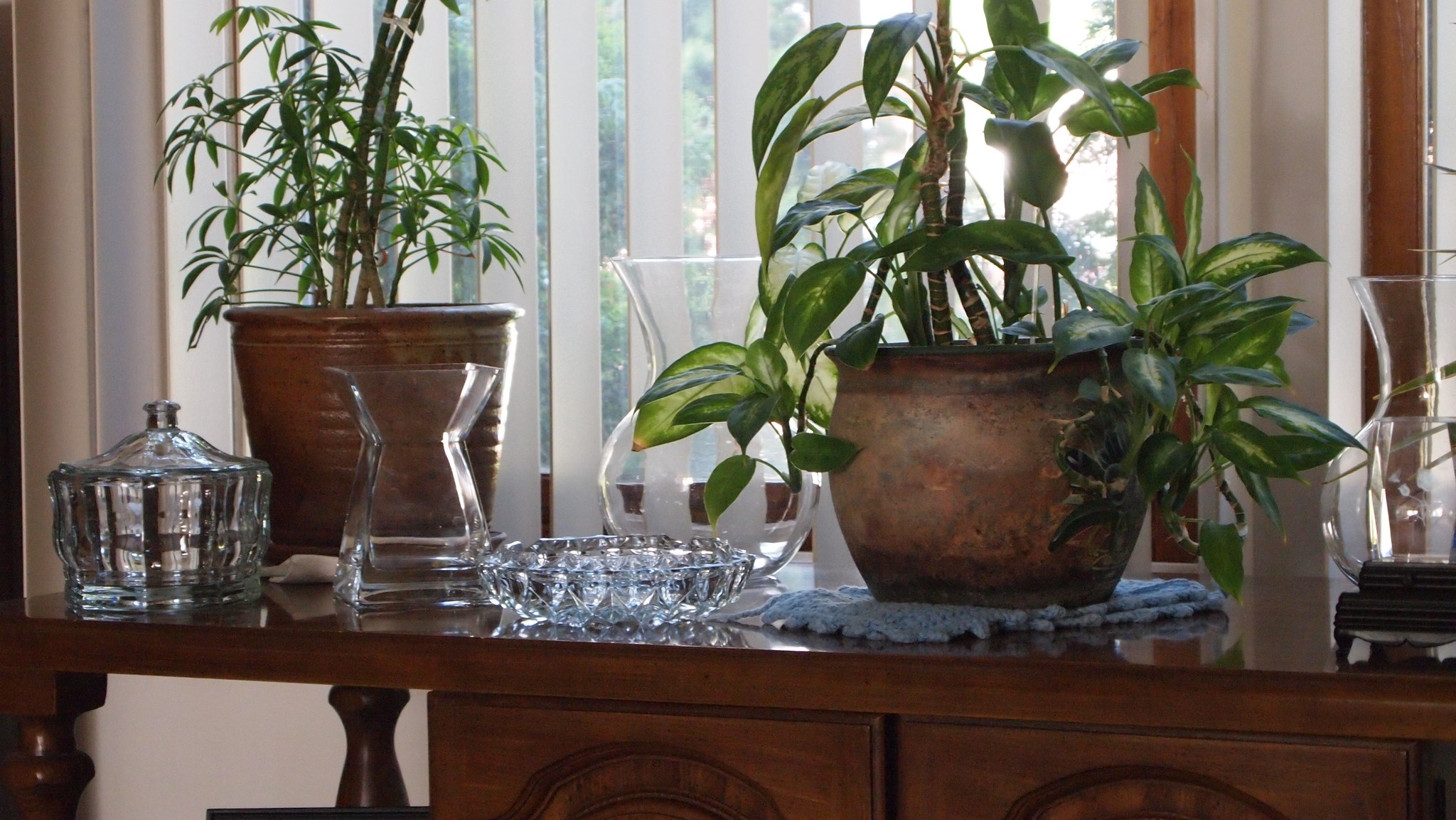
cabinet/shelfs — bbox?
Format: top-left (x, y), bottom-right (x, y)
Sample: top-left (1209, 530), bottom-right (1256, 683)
top-left (0, 550), bottom-right (1455, 819)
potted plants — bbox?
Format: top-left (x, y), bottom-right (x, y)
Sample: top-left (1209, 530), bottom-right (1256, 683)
top-left (629, 1), bottom-right (1370, 611)
top-left (154, 0), bottom-right (527, 566)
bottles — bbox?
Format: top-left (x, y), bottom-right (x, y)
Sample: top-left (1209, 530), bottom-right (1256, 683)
top-left (1322, 274), bottom-right (1456, 585)
top-left (598, 254), bottom-right (821, 579)
top-left (325, 362), bottom-right (504, 612)
